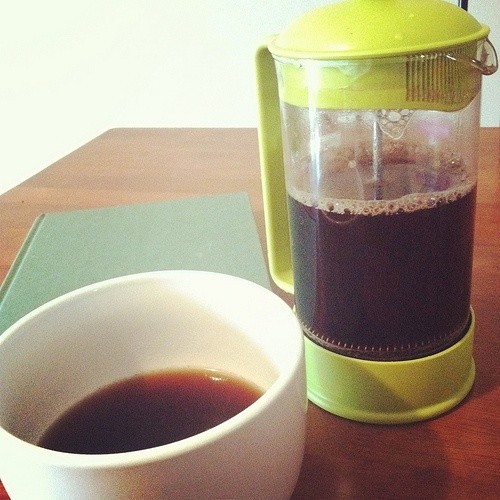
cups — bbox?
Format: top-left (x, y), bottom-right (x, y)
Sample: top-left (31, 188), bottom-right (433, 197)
top-left (0, 269), bottom-right (305, 500)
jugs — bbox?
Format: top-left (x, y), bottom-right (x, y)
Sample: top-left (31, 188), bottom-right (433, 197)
top-left (254, 2), bottom-right (497, 426)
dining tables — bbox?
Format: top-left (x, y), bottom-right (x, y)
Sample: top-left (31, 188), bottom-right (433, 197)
top-left (0, 125), bottom-right (500, 500)
